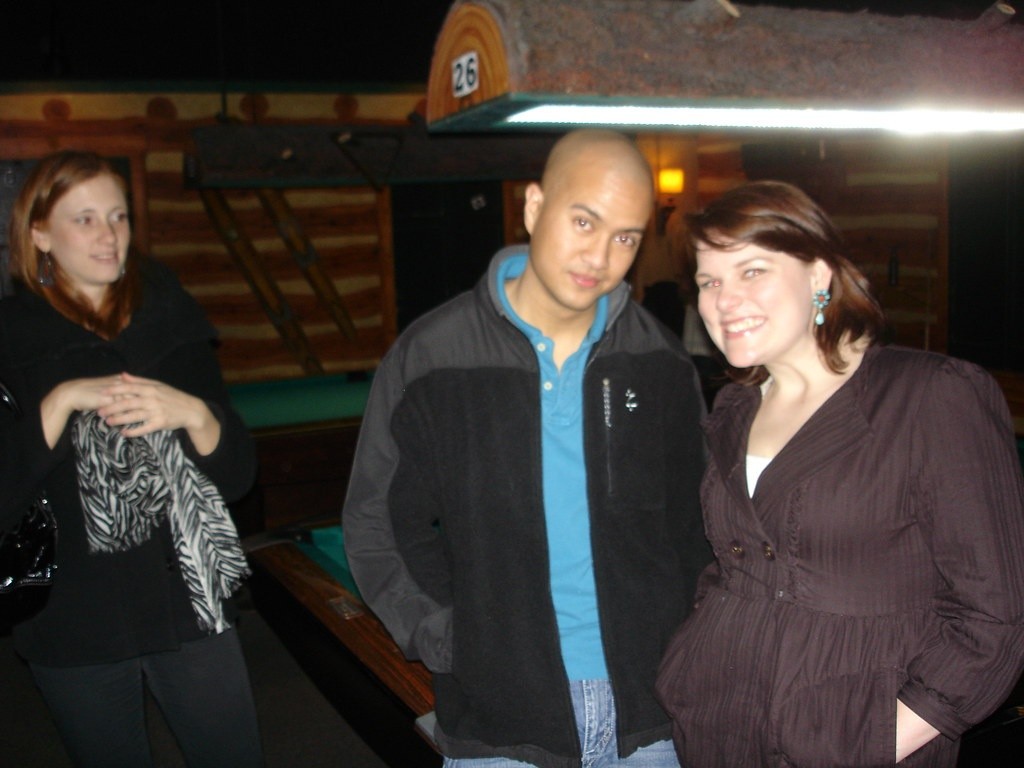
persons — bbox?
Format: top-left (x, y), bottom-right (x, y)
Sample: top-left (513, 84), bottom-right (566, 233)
top-left (652, 181), bottom-right (1024, 767)
top-left (0, 149), bottom-right (261, 768)
top-left (343, 128), bottom-right (709, 768)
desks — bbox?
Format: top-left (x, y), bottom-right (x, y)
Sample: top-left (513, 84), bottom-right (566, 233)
top-left (233, 520), bottom-right (447, 768)
top-left (221, 368), bottom-right (382, 497)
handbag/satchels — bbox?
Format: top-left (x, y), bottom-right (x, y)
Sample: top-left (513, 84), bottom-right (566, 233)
top-left (0, 385), bottom-right (58, 592)
